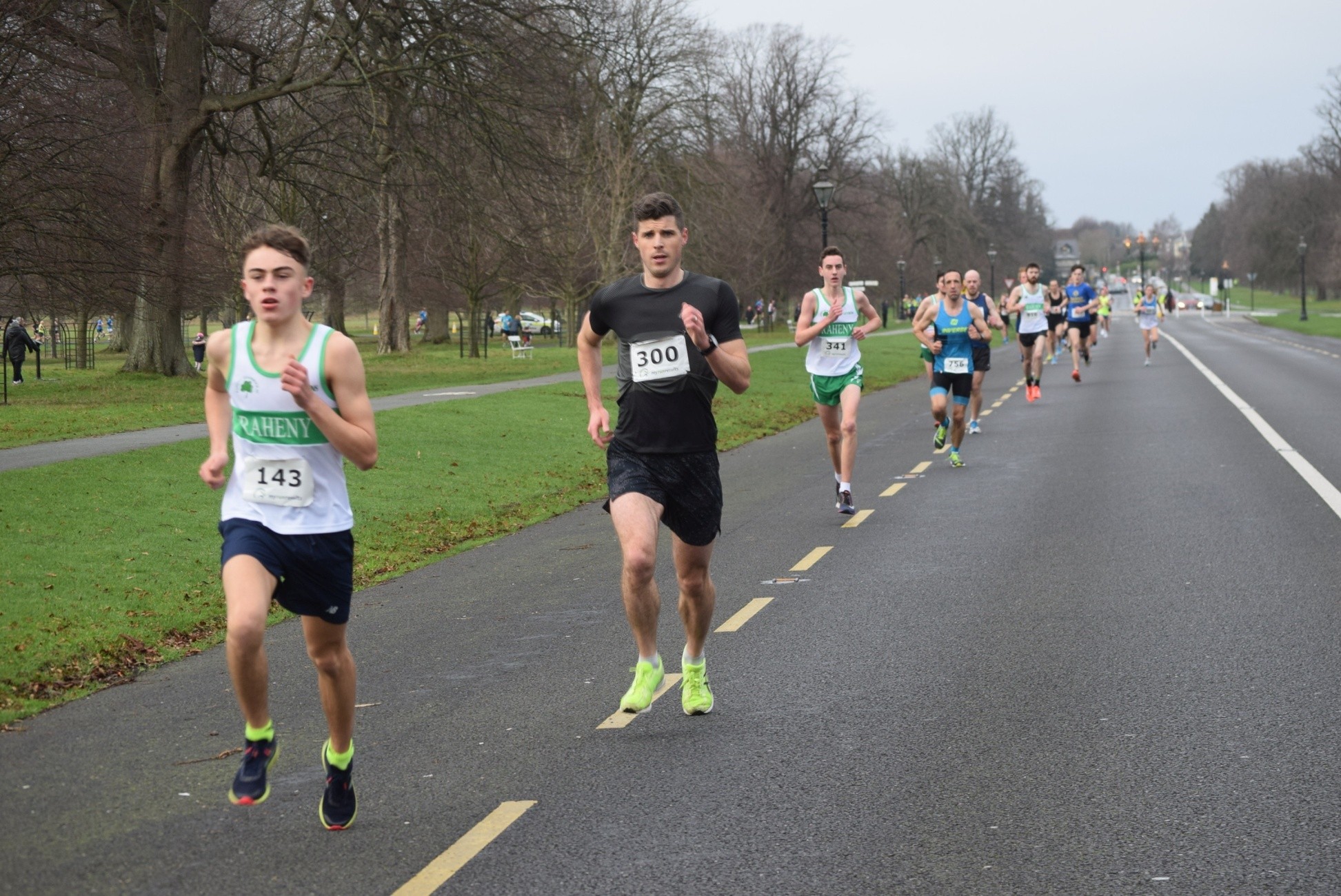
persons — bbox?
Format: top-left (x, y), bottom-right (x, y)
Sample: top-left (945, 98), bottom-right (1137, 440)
top-left (54, 318), bottom-right (62, 344)
top-left (795, 302), bottom-right (801, 320)
top-left (1134, 285), bottom-right (1165, 367)
top-left (5, 316), bottom-right (45, 384)
top-left (200, 222), bottom-right (378, 828)
top-left (1133, 285), bottom-right (1174, 324)
top-left (882, 299), bottom-right (890, 328)
top-left (487, 310), bottom-right (533, 347)
top-left (1003, 262), bottom-right (1113, 402)
top-left (912, 268), bottom-right (1004, 467)
top-left (997, 293), bottom-right (1010, 345)
top-left (414, 308), bottom-right (427, 334)
top-left (903, 292), bottom-right (929, 322)
top-left (192, 333), bottom-right (206, 371)
top-left (745, 298), bottom-right (775, 324)
top-left (576, 193), bottom-right (751, 717)
top-left (94, 316), bottom-right (114, 342)
top-left (796, 246), bottom-right (882, 514)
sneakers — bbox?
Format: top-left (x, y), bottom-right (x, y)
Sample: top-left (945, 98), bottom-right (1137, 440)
top-left (1032, 385), bottom-right (1041, 398)
top-left (678, 649), bottom-right (714, 716)
top-left (838, 490), bottom-right (855, 515)
top-left (933, 417), bottom-right (949, 448)
top-left (319, 739), bottom-right (359, 830)
top-left (1026, 385), bottom-right (1034, 401)
top-left (969, 418), bottom-right (980, 434)
top-left (228, 730), bottom-right (278, 805)
top-left (948, 451), bottom-right (965, 467)
top-left (835, 481), bottom-right (840, 502)
top-left (618, 654), bottom-right (666, 714)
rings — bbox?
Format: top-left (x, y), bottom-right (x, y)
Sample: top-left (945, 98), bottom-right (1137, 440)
top-left (695, 316), bottom-right (697, 320)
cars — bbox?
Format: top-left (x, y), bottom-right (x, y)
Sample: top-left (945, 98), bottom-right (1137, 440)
top-left (493, 311), bottom-right (561, 336)
top-left (1177, 295), bottom-right (1203, 311)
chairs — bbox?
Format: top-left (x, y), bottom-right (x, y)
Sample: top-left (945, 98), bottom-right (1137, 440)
top-left (507, 334), bottom-right (534, 360)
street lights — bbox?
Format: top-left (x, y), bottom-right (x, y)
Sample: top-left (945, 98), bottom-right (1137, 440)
top-left (987, 242), bottom-right (998, 303)
top-left (1295, 243), bottom-right (1308, 321)
top-left (1121, 232), bottom-right (1160, 313)
top-left (810, 163), bottom-right (836, 251)
top-left (896, 260), bottom-right (907, 321)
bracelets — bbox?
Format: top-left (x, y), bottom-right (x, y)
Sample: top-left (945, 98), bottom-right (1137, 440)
top-left (1162, 314), bottom-right (1164, 316)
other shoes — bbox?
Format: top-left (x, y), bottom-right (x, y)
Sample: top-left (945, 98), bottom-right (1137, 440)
top-left (1084, 352), bottom-right (1089, 362)
top-left (1071, 370), bottom-right (1081, 383)
top-left (1045, 339), bottom-right (1068, 364)
top-left (1145, 359), bottom-right (1151, 365)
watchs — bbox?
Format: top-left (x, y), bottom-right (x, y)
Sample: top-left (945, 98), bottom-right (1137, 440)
top-left (700, 334), bottom-right (718, 355)
top-left (979, 334), bottom-right (985, 341)
